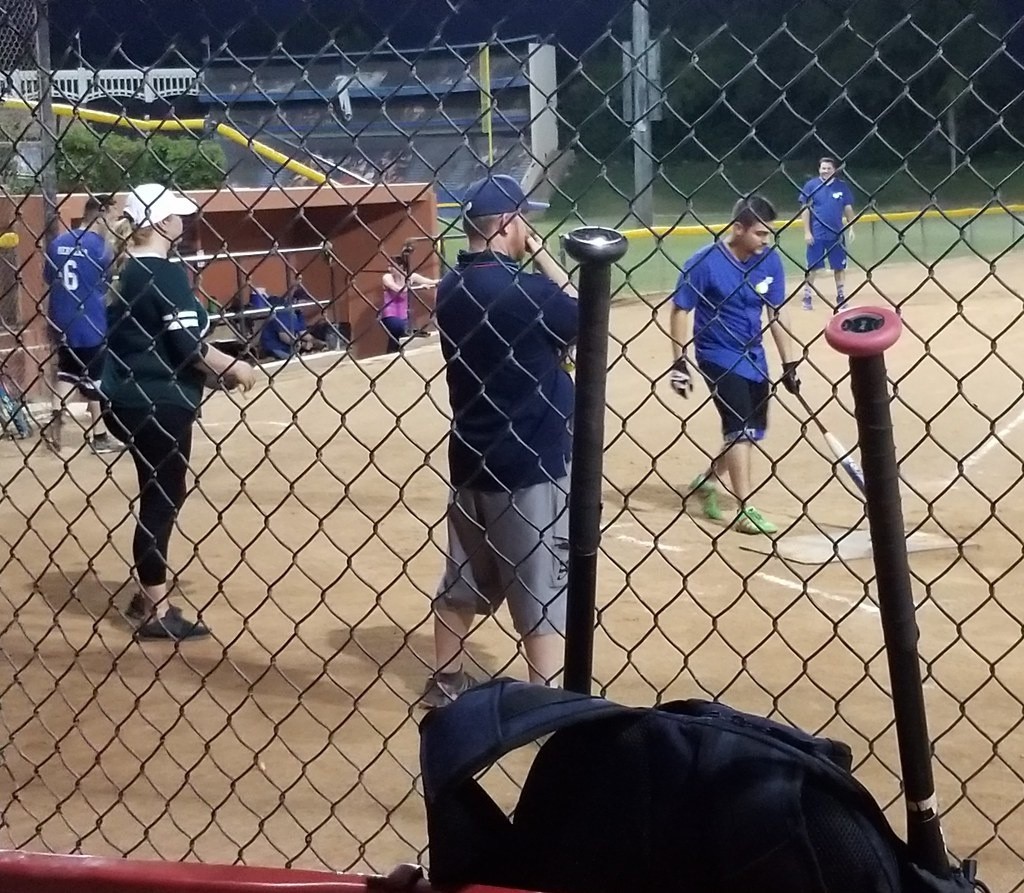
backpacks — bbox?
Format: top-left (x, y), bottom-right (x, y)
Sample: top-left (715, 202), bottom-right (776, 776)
top-left (416, 679), bottom-right (988, 892)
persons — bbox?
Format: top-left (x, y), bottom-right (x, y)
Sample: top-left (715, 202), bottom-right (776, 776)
top-left (100, 183), bottom-right (256, 642)
top-left (260, 288), bottom-right (329, 359)
top-left (798, 157), bottom-right (855, 310)
top-left (382, 256), bottom-right (441, 353)
top-left (419, 177), bottom-right (579, 709)
top-left (43, 194), bottom-right (127, 454)
top-left (670, 193), bottom-right (800, 534)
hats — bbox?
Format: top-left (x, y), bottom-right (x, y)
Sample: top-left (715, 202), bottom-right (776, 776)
top-left (461, 175), bottom-right (552, 217)
top-left (124, 184), bottom-right (198, 227)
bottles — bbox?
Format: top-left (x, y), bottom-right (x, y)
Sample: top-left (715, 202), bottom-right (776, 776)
top-left (208, 295), bottom-right (217, 313)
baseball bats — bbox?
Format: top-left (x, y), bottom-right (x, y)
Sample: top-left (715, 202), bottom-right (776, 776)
top-left (560, 224), bottom-right (630, 697)
top-left (796, 388), bottom-right (864, 496)
top-left (825, 304), bottom-right (954, 879)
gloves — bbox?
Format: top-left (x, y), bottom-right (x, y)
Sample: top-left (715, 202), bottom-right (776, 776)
top-left (669, 360), bottom-right (694, 401)
top-left (781, 362), bottom-right (802, 396)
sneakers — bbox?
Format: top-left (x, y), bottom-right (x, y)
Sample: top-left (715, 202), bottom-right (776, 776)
top-left (735, 507), bottom-right (778, 535)
top-left (687, 474), bottom-right (726, 521)
top-left (801, 297), bottom-right (813, 310)
top-left (127, 592), bottom-right (183, 621)
top-left (91, 435), bottom-right (126, 454)
top-left (140, 609), bottom-right (212, 639)
top-left (42, 417), bottom-right (66, 452)
top-left (836, 294), bottom-right (849, 310)
top-left (418, 674), bottom-right (483, 708)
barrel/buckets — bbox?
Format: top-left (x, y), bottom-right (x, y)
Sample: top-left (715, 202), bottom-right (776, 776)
top-left (249, 286), bottom-right (270, 308)
